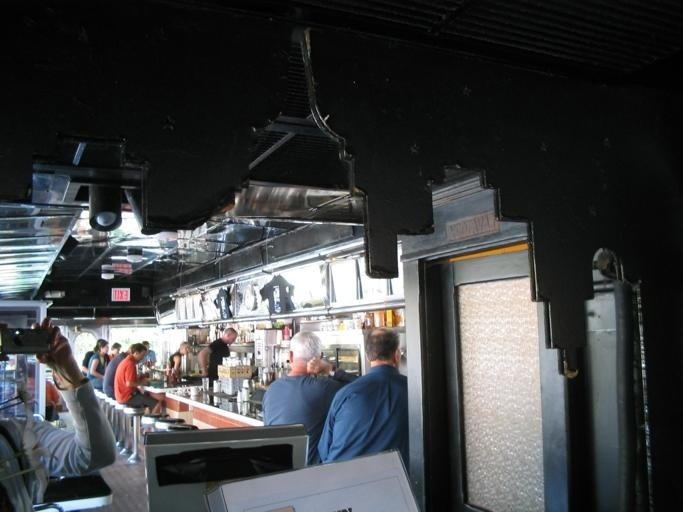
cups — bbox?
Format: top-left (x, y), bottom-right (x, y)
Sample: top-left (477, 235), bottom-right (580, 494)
top-left (187, 377), bottom-right (221, 397)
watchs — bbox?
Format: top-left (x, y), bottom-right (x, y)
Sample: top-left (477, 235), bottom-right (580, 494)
top-left (327, 367), bottom-right (337, 379)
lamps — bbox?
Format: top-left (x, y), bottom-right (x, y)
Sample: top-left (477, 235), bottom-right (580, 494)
top-left (88, 186), bottom-right (123, 232)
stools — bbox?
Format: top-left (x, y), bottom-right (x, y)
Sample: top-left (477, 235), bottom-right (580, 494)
top-left (94, 388), bottom-right (197, 464)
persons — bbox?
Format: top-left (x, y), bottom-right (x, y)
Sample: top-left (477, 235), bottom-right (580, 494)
top-left (167, 340), bottom-right (192, 380)
top-left (79, 337), bottom-right (161, 437)
top-left (0, 315), bottom-right (118, 511)
top-left (198, 327), bottom-right (240, 387)
top-left (260, 330), bottom-right (359, 467)
top-left (25, 360), bottom-right (61, 421)
top-left (317, 324), bottom-right (408, 463)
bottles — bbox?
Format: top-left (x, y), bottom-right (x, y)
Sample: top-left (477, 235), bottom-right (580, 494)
top-left (282, 326), bottom-right (290, 340)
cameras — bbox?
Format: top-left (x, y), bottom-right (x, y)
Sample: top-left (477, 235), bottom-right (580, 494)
top-left (1, 328), bottom-right (48, 354)
